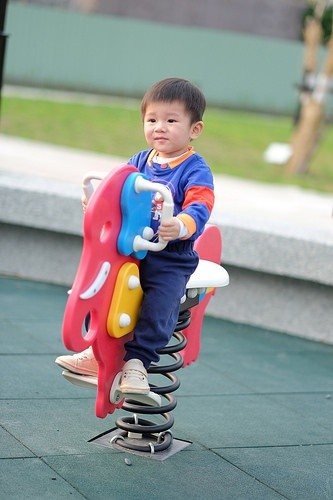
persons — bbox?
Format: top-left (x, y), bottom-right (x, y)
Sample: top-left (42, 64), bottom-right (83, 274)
top-left (54, 78), bottom-right (215, 395)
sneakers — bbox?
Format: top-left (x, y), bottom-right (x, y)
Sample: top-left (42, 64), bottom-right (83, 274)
top-left (53, 347), bottom-right (101, 376)
top-left (120, 357), bottom-right (152, 394)
